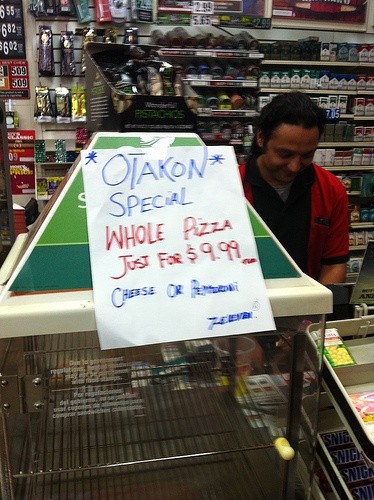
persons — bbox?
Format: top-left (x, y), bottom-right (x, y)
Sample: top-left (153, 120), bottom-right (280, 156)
top-left (234, 92), bottom-right (351, 322)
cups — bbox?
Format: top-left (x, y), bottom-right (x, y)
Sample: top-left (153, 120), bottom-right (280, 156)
top-left (214, 337), bottom-right (256, 397)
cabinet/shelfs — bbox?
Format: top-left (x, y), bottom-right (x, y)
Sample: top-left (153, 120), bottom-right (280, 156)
top-left (80, 28), bottom-right (374, 281)
